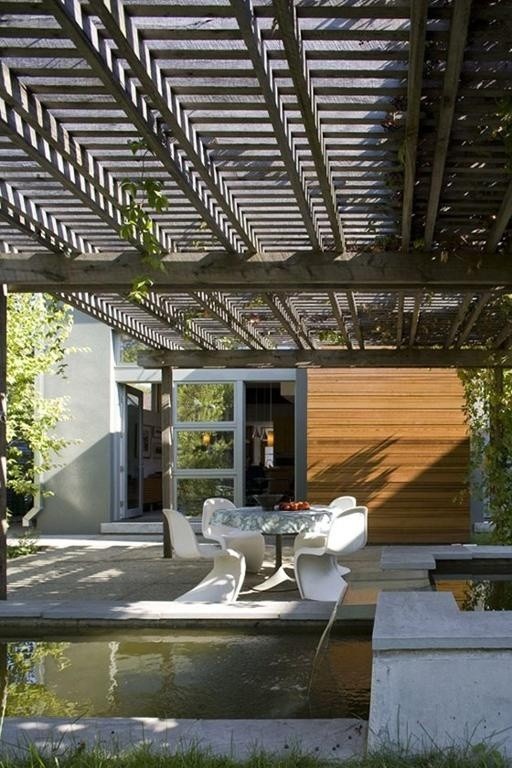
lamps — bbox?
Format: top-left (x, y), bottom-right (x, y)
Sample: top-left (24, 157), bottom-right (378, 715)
top-left (267, 383), bottom-right (275, 446)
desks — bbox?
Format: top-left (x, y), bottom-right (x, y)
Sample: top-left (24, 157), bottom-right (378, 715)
top-left (255, 477), bottom-right (290, 493)
top-left (212, 505), bottom-right (331, 593)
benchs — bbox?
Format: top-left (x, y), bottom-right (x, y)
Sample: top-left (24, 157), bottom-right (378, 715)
top-left (143, 498), bottom-right (162, 512)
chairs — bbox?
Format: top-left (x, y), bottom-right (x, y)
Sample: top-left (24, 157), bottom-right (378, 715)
top-left (292, 506), bottom-right (369, 603)
top-left (294, 496), bottom-right (357, 577)
top-left (161, 509), bottom-right (246, 602)
top-left (246, 477), bottom-right (264, 494)
top-left (202, 497), bottom-right (265, 573)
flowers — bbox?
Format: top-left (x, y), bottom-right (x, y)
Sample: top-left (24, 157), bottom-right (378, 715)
top-left (268, 465), bottom-right (281, 472)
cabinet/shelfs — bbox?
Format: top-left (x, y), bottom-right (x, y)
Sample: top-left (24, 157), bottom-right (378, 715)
top-left (143, 478), bottom-right (162, 497)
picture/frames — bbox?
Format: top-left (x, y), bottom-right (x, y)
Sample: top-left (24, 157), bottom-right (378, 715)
top-left (136, 424), bottom-right (152, 459)
top-left (151, 442), bottom-right (162, 458)
top-left (153, 426), bottom-right (161, 438)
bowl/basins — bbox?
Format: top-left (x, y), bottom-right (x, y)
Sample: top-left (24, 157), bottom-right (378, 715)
top-left (252, 492), bottom-right (286, 512)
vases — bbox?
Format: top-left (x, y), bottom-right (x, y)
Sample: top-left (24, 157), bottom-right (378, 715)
top-left (272, 471), bottom-right (276, 478)
